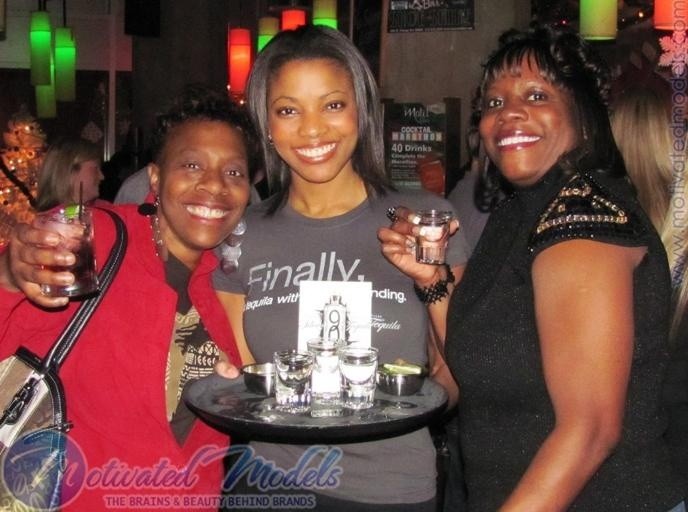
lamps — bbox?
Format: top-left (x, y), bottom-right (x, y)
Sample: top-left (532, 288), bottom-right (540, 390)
top-left (312, 0), bottom-right (338, 30)
top-left (28, 0), bottom-right (77, 119)
top-left (227, 0), bottom-right (252, 105)
top-left (579, 0), bottom-right (617, 40)
top-left (653, 0), bottom-right (688, 30)
top-left (257, 0), bottom-right (280, 52)
top-left (281, 0), bottom-right (306, 31)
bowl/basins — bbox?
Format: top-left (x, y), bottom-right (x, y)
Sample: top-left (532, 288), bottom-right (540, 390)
top-left (377, 361), bottom-right (427, 396)
top-left (241, 361), bottom-right (275, 398)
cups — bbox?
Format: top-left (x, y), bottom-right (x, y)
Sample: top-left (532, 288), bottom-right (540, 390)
top-left (306, 336), bottom-right (348, 418)
top-left (414, 210), bottom-right (451, 264)
top-left (273, 350), bottom-right (316, 414)
top-left (338, 344), bottom-right (379, 411)
top-left (35, 212), bottom-right (101, 302)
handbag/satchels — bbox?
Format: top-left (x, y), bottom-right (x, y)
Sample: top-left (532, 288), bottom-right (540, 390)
top-left (0, 344), bottom-right (69, 511)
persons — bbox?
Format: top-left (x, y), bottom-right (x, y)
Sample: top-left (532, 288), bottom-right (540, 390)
top-left (609, 69), bottom-right (688, 334)
top-left (0, 93), bottom-right (263, 511)
top-left (35, 137), bottom-right (105, 212)
top-left (112, 163), bottom-right (263, 208)
top-left (378, 24), bottom-right (688, 511)
top-left (212, 24), bottom-right (470, 511)
top-left (447, 111), bottom-right (506, 261)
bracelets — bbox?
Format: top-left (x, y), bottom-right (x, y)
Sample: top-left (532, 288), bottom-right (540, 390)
top-left (414, 263), bottom-right (456, 305)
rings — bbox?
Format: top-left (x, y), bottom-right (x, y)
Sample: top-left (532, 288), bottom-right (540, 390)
top-left (387, 206), bottom-right (398, 222)
